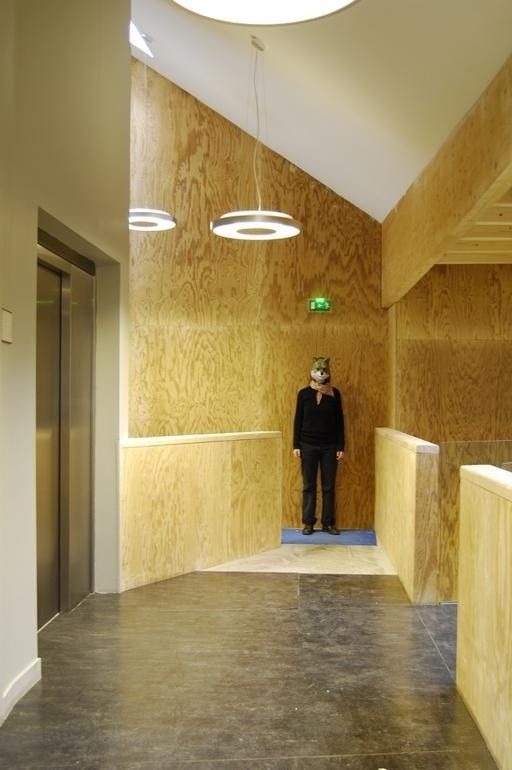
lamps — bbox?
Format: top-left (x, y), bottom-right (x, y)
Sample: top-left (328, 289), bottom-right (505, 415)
top-left (128, 32), bottom-right (178, 233)
top-left (206, 33), bottom-right (305, 242)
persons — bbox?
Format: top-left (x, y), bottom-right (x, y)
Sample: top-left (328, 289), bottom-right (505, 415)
top-left (293, 357), bottom-right (345, 535)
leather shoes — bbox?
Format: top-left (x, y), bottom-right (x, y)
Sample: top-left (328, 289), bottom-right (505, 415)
top-left (323, 525), bottom-right (340, 535)
top-left (303, 524), bottom-right (314, 535)
